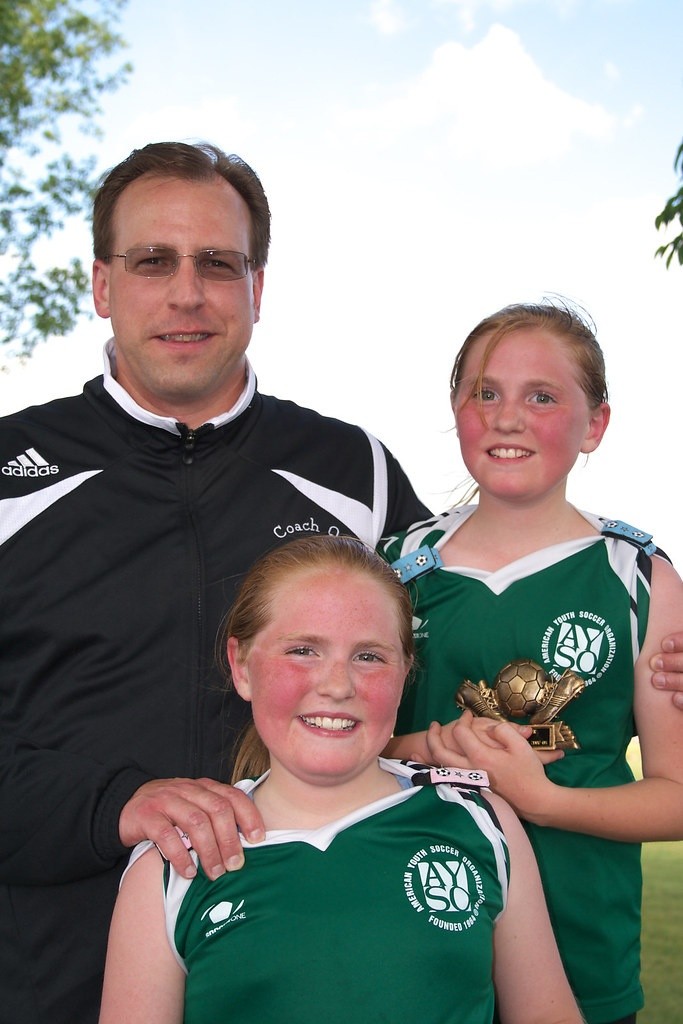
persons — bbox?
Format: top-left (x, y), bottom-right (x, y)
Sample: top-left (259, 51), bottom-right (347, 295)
top-left (97, 536), bottom-right (581, 1023)
top-left (0, 142), bottom-right (683, 1024)
top-left (383, 304), bottom-right (683, 1024)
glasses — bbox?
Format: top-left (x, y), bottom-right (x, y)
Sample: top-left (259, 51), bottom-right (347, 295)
top-left (105, 247), bottom-right (256, 281)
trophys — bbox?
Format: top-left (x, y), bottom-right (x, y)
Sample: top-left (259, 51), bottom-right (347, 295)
top-left (454, 661), bottom-right (585, 751)
